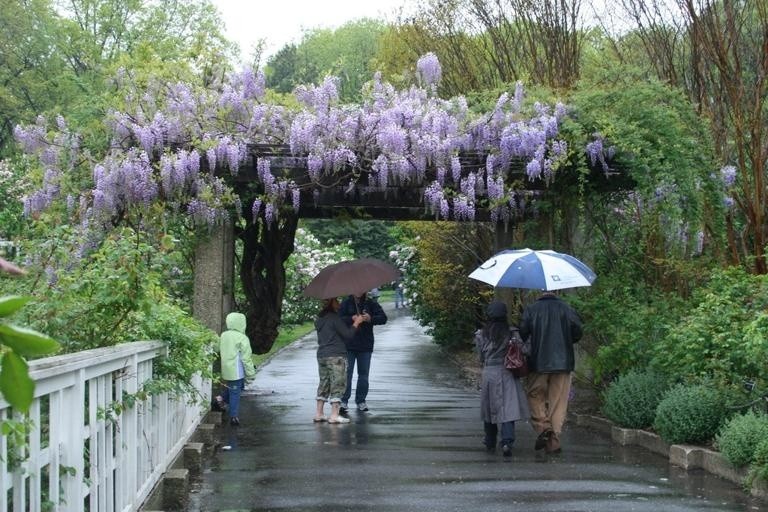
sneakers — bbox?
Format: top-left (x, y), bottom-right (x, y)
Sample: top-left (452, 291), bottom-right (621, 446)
top-left (356, 403), bottom-right (368, 411)
top-left (502, 445), bottom-right (512, 457)
top-left (482, 437), bottom-right (495, 448)
top-left (534, 431), bottom-right (550, 450)
top-left (339, 403), bottom-right (348, 411)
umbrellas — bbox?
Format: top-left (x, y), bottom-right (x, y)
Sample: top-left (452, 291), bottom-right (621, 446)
top-left (468, 247), bottom-right (598, 302)
top-left (303, 258), bottom-right (402, 304)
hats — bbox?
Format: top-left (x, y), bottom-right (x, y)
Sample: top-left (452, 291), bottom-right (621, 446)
top-left (323, 298), bottom-right (331, 308)
top-left (487, 302), bottom-right (508, 313)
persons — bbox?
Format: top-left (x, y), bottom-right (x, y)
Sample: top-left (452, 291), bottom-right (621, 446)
top-left (392, 281), bottom-right (405, 309)
top-left (313, 298), bottom-right (364, 424)
top-left (474, 301), bottom-right (528, 457)
top-left (339, 290), bottom-right (388, 412)
top-left (211, 312), bottom-right (255, 425)
top-left (517, 290), bottom-right (583, 453)
top-left (369, 287), bottom-right (381, 303)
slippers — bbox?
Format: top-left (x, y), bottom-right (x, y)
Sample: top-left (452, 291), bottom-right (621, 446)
top-left (313, 414), bottom-right (328, 422)
top-left (328, 415), bottom-right (349, 424)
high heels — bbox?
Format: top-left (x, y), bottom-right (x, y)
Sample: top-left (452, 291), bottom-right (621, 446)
top-left (213, 396), bottom-right (226, 411)
top-left (231, 417), bottom-right (240, 425)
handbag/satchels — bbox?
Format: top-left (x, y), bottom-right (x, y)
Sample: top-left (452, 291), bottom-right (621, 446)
top-left (213, 352), bottom-right (221, 373)
top-left (504, 337), bottom-right (528, 377)
